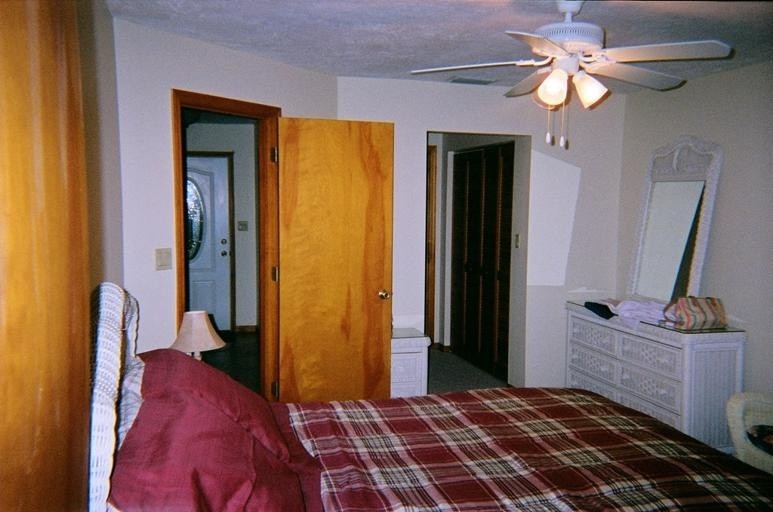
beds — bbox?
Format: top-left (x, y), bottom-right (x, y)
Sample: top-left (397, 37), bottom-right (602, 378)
top-left (88, 282), bottom-right (773, 512)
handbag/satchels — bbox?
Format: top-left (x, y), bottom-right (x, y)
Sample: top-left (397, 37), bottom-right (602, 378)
top-left (674, 298), bottom-right (727, 330)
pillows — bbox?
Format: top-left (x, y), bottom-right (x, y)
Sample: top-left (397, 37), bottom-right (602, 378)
top-left (748, 424), bottom-right (773, 457)
top-left (107, 350), bottom-right (302, 511)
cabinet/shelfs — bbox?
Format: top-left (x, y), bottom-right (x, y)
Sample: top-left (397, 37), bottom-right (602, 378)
top-left (564, 301), bottom-right (745, 455)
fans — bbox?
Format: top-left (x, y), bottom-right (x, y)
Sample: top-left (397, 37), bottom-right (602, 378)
top-left (411, 0), bottom-right (730, 97)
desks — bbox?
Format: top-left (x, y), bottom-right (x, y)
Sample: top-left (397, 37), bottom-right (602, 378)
top-left (391, 328), bottom-right (431, 397)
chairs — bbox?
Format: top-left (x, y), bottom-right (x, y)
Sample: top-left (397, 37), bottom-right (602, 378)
top-left (728, 391), bottom-right (773, 474)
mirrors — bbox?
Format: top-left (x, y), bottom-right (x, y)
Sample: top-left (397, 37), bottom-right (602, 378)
top-left (628, 133), bottom-right (726, 310)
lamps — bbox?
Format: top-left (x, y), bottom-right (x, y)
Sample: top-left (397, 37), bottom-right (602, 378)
top-left (169, 310), bottom-right (227, 361)
top-left (537, 57), bottom-right (608, 108)
